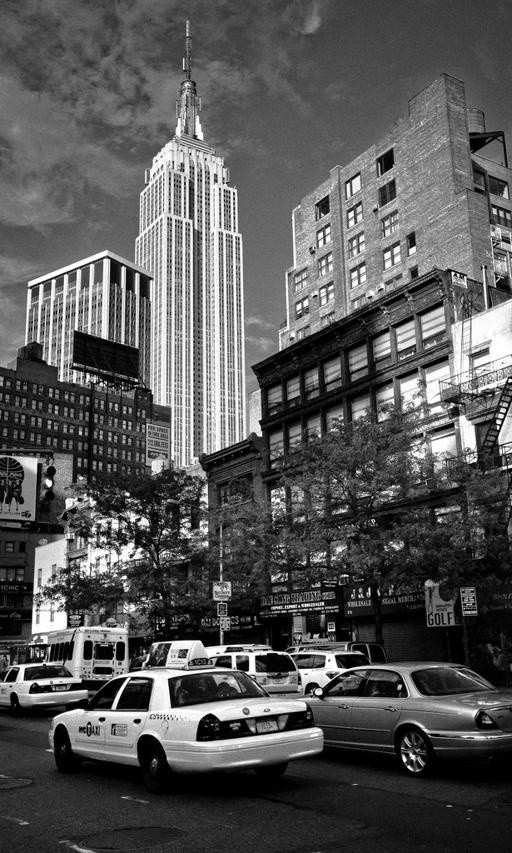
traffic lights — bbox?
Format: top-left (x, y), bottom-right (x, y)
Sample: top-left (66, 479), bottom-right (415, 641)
top-left (42, 464), bottom-right (55, 504)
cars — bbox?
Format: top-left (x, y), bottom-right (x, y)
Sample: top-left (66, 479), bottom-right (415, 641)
top-left (0, 662), bottom-right (89, 716)
top-left (294, 661), bottom-right (512, 778)
top-left (47, 639), bottom-right (325, 794)
